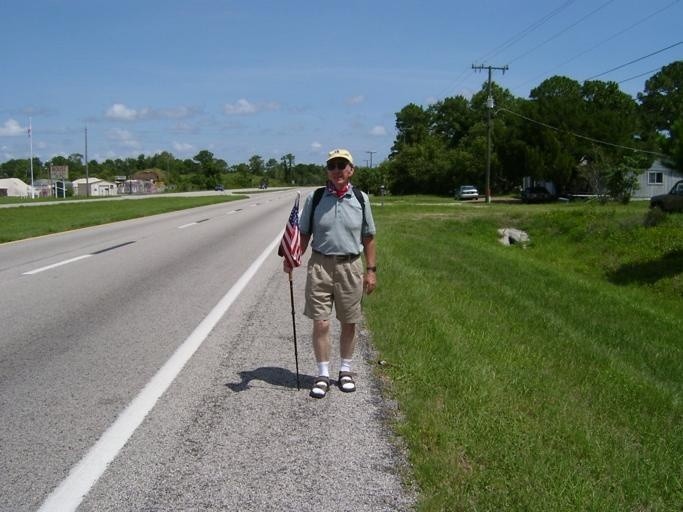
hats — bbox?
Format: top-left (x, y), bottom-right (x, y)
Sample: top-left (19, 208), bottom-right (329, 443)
top-left (326, 149), bottom-right (352, 164)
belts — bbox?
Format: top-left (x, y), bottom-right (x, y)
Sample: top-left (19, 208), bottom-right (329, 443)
top-left (312, 248), bottom-right (356, 257)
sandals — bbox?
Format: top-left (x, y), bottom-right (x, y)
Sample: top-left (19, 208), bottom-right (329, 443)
top-left (310, 376), bottom-right (330, 397)
top-left (339, 371), bottom-right (356, 392)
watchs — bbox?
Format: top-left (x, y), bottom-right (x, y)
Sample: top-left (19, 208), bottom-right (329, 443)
top-left (366, 264), bottom-right (376, 272)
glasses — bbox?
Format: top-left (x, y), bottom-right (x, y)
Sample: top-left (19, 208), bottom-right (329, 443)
top-left (328, 162), bottom-right (348, 170)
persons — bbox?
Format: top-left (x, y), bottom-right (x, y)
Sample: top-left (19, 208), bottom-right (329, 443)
top-left (284, 149), bottom-right (376, 398)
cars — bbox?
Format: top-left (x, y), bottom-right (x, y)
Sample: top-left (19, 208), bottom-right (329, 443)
top-left (454, 185), bottom-right (479, 201)
top-left (649, 180), bottom-right (683, 213)
top-left (521, 187), bottom-right (551, 203)
top-left (259, 183), bottom-right (267, 190)
top-left (214, 185), bottom-right (226, 192)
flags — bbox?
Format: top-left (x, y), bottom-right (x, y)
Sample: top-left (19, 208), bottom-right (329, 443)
top-left (278, 193), bottom-right (302, 272)
